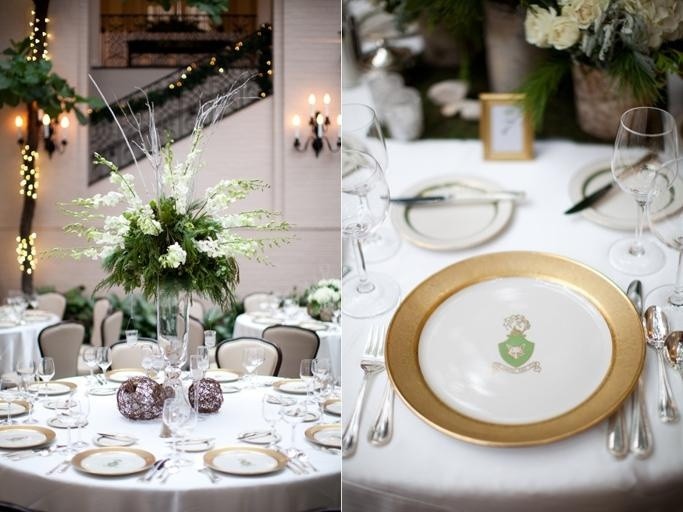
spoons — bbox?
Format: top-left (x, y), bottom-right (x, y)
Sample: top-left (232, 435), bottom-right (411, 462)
top-left (663, 330), bottom-right (683, 382)
top-left (642, 305), bottom-right (680, 423)
top-left (162, 465), bottom-right (179, 484)
top-left (626, 279), bottom-right (653, 460)
top-left (46, 452), bottom-right (74, 475)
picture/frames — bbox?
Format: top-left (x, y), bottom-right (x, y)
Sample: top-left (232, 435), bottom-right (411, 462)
top-left (479, 92), bottom-right (534, 161)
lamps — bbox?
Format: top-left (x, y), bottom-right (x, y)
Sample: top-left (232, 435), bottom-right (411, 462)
top-left (15, 114), bottom-right (69, 160)
top-left (293, 92), bottom-right (341, 157)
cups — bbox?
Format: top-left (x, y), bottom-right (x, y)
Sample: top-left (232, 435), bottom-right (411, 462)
top-left (384, 89), bottom-right (423, 141)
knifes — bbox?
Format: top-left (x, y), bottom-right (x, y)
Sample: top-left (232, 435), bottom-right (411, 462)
top-left (278, 443), bottom-right (321, 478)
top-left (97, 432), bottom-right (140, 442)
top-left (566, 151), bottom-right (659, 213)
top-left (145, 458), bottom-right (170, 483)
top-left (137, 457), bottom-right (162, 483)
top-left (386, 189), bottom-right (527, 203)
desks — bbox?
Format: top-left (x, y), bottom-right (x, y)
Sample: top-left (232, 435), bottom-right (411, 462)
top-left (0, 287), bottom-right (341, 512)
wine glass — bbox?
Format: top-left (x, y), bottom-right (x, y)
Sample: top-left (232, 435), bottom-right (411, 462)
top-left (342, 102), bottom-right (398, 261)
top-left (1, 357), bottom-right (55, 423)
top-left (140, 335), bottom-right (165, 376)
top-left (299, 358), bottom-right (338, 403)
top-left (341, 148), bottom-right (399, 319)
top-left (0, 290), bottom-right (40, 325)
top-left (257, 295), bottom-right (304, 320)
top-left (241, 345), bottom-right (266, 392)
top-left (260, 388), bottom-right (313, 453)
top-left (645, 158), bottom-right (683, 332)
top-left (164, 396), bottom-right (196, 467)
top-left (82, 346), bottom-right (112, 386)
top-left (609, 106), bottom-right (679, 276)
top-left (189, 330), bottom-right (216, 415)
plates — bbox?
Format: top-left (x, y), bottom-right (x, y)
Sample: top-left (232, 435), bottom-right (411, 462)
top-left (88, 386), bottom-right (117, 395)
top-left (72, 447), bottom-right (154, 476)
top-left (303, 411), bottom-right (318, 421)
top-left (48, 414), bottom-right (89, 429)
top-left (568, 156), bottom-right (682, 229)
top-left (45, 397), bottom-right (77, 409)
top-left (26, 380), bottom-right (77, 396)
top-left (221, 384), bottom-right (240, 393)
top-left (0, 399), bottom-right (32, 417)
top-left (94, 433), bottom-right (134, 446)
top-left (203, 368), bottom-right (243, 383)
top-left (271, 378), bottom-right (323, 393)
top-left (297, 320), bottom-right (328, 331)
top-left (203, 447), bottom-right (288, 475)
top-left (389, 177), bottom-right (513, 250)
top-left (104, 368), bottom-right (159, 381)
top-left (0, 425), bottom-right (55, 450)
top-left (320, 399), bottom-right (341, 416)
top-left (253, 316), bottom-right (284, 324)
top-left (383, 249), bottom-right (646, 445)
top-left (305, 422), bottom-right (340, 448)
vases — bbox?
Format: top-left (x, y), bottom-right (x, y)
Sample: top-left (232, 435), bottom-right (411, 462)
top-left (571, 56), bottom-right (649, 143)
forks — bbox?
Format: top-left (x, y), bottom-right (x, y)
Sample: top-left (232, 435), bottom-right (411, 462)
top-left (342, 323), bottom-right (388, 459)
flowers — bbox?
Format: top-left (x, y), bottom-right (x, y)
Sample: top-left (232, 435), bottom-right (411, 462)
top-left (36, 73), bottom-right (296, 331)
top-left (522, 0), bottom-right (683, 131)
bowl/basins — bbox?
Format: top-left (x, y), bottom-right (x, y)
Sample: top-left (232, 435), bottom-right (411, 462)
top-left (429, 81), bottom-right (468, 104)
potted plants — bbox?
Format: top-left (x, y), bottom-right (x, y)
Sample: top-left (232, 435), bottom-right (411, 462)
top-left (389, 0), bottom-right (564, 108)
